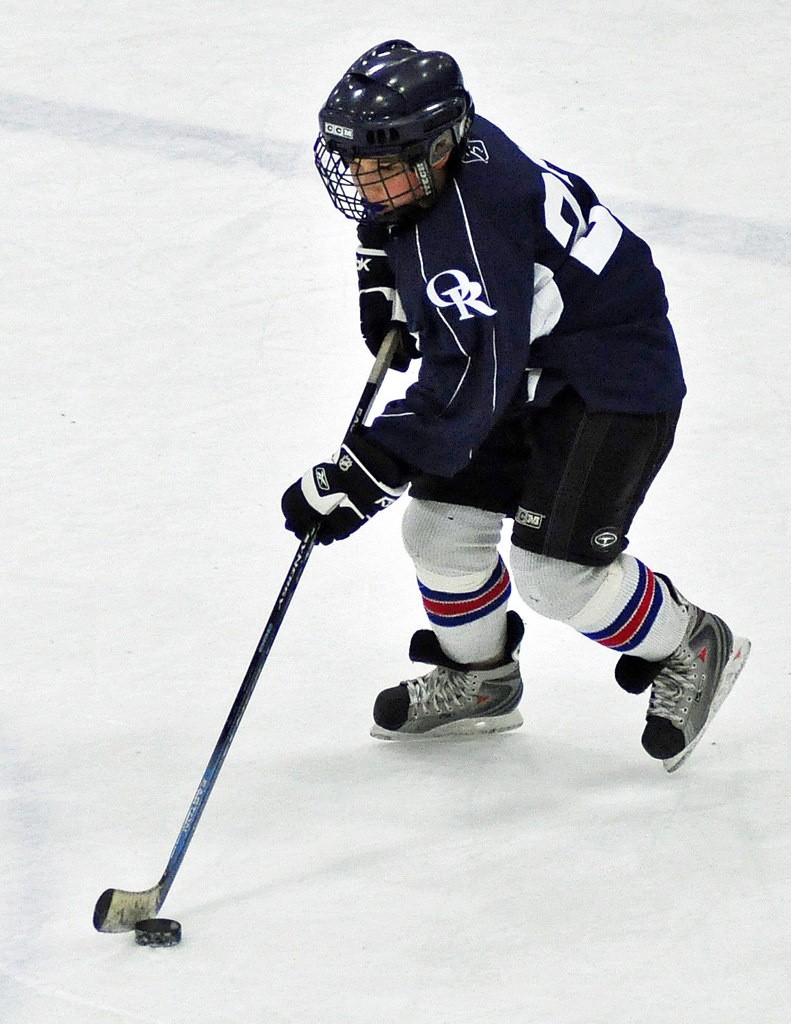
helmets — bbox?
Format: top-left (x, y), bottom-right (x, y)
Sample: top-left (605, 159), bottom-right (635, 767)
top-left (314, 40), bottom-right (475, 237)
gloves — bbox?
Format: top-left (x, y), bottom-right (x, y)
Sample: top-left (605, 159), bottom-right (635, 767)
top-left (355, 245), bottom-right (423, 373)
top-left (281, 424), bottom-right (413, 546)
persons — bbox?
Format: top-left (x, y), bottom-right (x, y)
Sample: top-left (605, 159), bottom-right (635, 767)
top-left (279, 37), bottom-right (752, 773)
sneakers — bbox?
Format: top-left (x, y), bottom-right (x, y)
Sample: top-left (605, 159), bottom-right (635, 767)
top-left (615, 573), bottom-right (751, 773)
top-left (369, 610), bottom-right (525, 740)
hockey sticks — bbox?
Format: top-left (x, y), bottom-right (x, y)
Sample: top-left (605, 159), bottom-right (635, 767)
top-left (90, 289), bottom-right (404, 934)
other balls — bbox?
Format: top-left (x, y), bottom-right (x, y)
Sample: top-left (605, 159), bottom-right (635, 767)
top-left (131, 918), bottom-right (185, 949)
